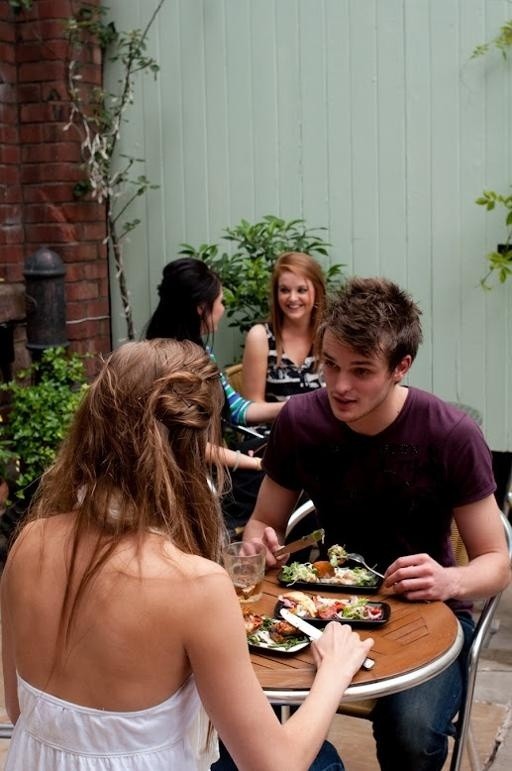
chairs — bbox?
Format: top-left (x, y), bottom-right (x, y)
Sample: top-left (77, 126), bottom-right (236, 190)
top-left (203, 473), bottom-right (248, 543)
top-left (489, 448), bottom-right (512, 516)
top-left (216, 361), bottom-right (274, 458)
top-left (274, 505), bottom-right (512, 771)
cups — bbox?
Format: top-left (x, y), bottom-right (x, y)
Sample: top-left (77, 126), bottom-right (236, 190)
top-left (221, 539), bottom-right (267, 603)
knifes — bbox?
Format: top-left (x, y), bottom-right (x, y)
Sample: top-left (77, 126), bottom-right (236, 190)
top-left (273, 528), bottom-right (325, 559)
top-left (279, 607), bottom-right (376, 670)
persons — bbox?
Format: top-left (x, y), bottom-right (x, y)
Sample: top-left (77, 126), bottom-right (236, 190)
top-left (1, 336), bottom-right (374, 771)
top-left (241, 250), bottom-right (327, 457)
top-left (139, 256), bottom-right (285, 524)
top-left (239, 275), bottom-right (512, 771)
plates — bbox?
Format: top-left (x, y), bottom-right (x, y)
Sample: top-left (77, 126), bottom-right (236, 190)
top-left (273, 594), bottom-right (391, 625)
top-left (277, 561), bottom-right (386, 591)
top-left (244, 615), bottom-right (311, 657)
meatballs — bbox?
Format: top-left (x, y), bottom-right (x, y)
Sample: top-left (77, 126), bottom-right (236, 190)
top-left (313, 561), bottom-right (335, 579)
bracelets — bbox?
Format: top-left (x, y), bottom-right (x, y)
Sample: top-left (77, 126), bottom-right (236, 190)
top-left (255, 456), bottom-right (261, 471)
top-left (232, 448), bottom-right (241, 472)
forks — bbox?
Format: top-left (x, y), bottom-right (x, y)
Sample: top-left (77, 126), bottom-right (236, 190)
top-left (336, 550), bottom-right (432, 605)
